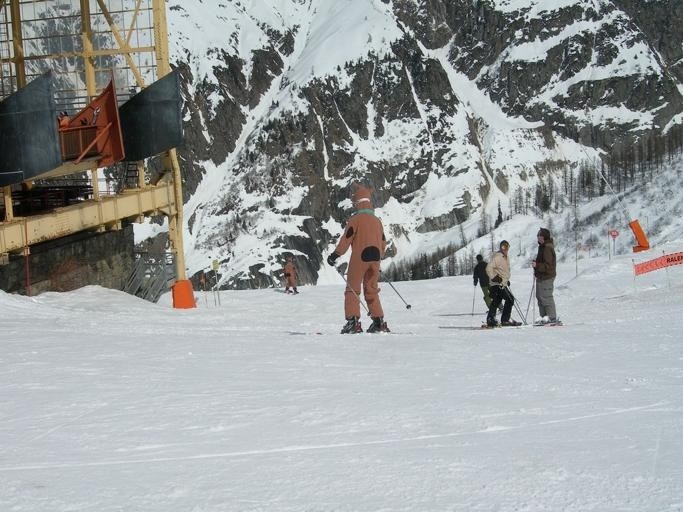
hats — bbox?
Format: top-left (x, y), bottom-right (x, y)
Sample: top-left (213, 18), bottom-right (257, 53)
top-left (536, 229), bottom-right (549, 241)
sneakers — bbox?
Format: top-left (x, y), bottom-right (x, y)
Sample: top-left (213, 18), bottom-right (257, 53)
top-left (502, 321), bottom-right (511, 325)
top-left (341, 316), bottom-right (357, 333)
top-left (535, 318), bottom-right (555, 324)
top-left (486, 318), bottom-right (498, 325)
top-left (366, 317), bottom-right (383, 332)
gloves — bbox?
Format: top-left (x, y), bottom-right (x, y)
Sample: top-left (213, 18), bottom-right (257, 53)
top-left (326, 252), bottom-right (339, 266)
top-left (491, 275), bottom-right (501, 283)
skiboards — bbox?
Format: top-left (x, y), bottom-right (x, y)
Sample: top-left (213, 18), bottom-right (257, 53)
top-left (440, 320), bottom-right (584, 330)
top-left (287, 327), bottom-right (415, 336)
top-left (285, 290), bottom-right (299, 296)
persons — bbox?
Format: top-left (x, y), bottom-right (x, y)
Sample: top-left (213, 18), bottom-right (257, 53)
top-left (284, 257), bottom-right (300, 295)
top-left (472, 255), bottom-right (504, 314)
top-left (531, 228), bottom-right (557, 324)
top-left (485, 240), bottom-right (517, 325)
top-left (327, 187), bottom-right (386, 334)
